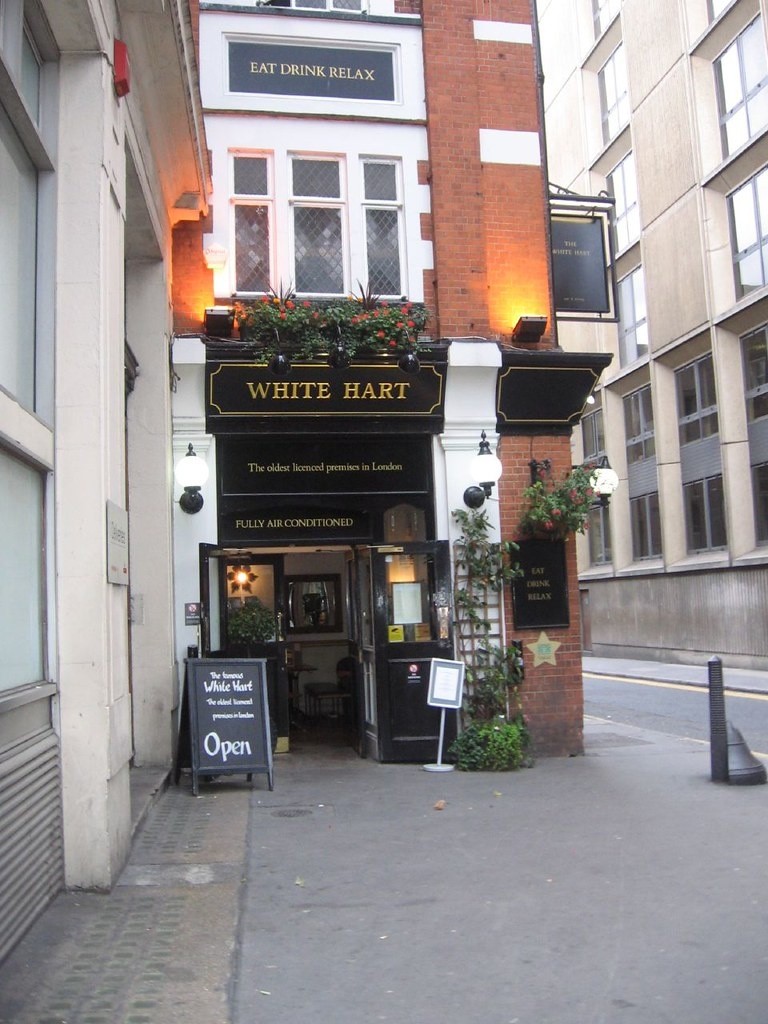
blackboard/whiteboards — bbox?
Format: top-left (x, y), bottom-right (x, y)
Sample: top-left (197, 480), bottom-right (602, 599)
top-left (183, 656), bottom-right (274, 776)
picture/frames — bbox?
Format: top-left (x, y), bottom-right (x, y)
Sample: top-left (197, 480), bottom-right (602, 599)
top-left (277, 574), bottom-right (342, 634)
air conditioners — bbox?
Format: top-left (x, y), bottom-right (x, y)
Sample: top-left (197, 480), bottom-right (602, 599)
top-left (175, 443), bottom-right (208, 515)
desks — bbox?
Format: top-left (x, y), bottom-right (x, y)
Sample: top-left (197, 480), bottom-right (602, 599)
top-left (288, 664), bottom-right (319, 723)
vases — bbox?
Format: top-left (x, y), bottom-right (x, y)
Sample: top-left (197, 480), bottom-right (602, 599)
top-left (531, 522), bottom-right (566, 543)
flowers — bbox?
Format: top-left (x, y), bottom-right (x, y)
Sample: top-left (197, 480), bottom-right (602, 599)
top-left (512, 461), bottom-right (600, 543)
top-left (244, 279), bottom-right (433, 363)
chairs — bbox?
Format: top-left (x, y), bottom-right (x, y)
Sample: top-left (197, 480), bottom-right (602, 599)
top-left (304, 656), bottom-right (357, 743)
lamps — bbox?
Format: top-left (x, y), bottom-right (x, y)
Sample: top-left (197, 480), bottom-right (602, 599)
top-left (511, 317), bottom-right (548, 344)
top-left (577, 457), bottom-right (618, 511)
top-left (463, 430), bottom-right (502, 508)
top-left (322, 324), bottom-right (352, 369)
top-left (203, 307), bottom-right (237, 338)
top-left (269, 327), bottom-right (292, 375)
top-left (395, 328), bottom-right (422, 376)
